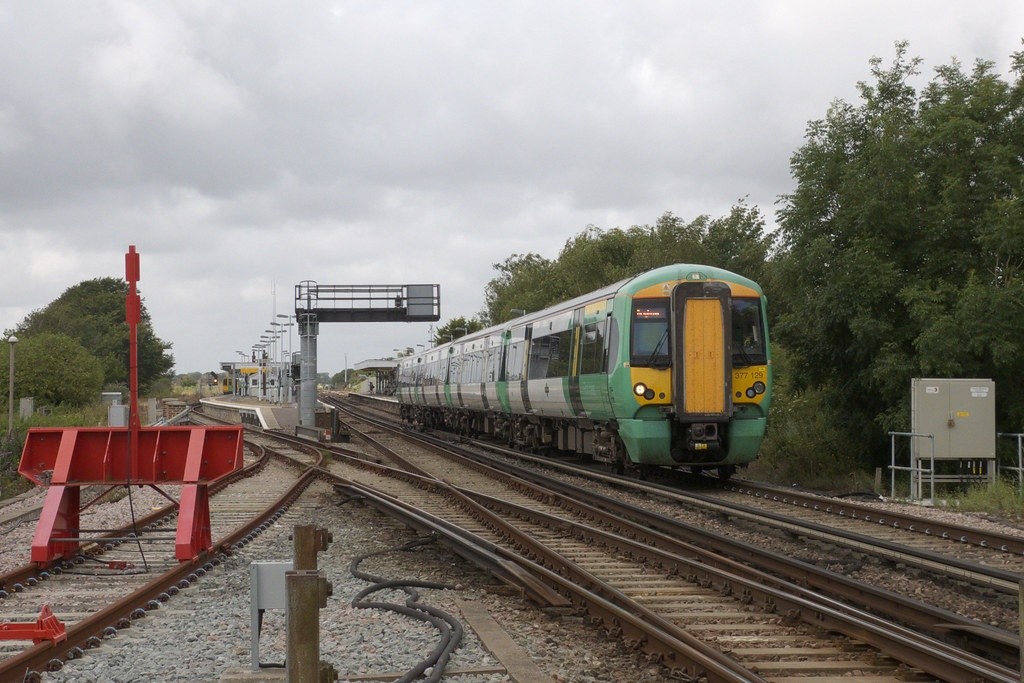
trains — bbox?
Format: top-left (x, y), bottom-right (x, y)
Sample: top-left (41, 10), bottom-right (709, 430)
top-left (394, 262), bottom-right (773, 485)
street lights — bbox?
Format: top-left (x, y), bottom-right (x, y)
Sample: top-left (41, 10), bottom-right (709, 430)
top-left (393, 349), bottom-right (404, 358)
top-left (442, 334), bottom-right (453, 342)
top-left (417, 344), bottom-right (426, 351)
top-left (510, 309), bottom-right (526, 316)
top-left (454, 326), bottom-right (468, 336)
top-left (478, 319), bottom-right (491, 327)
top-left (6, 336), bottom-right (20, 444)
top-left (234, 322), bottom-right (295, 400)
top-left (276, 313), bottom-right (298, 402)
top-left (427, 340), bottom-right (438, 347)
